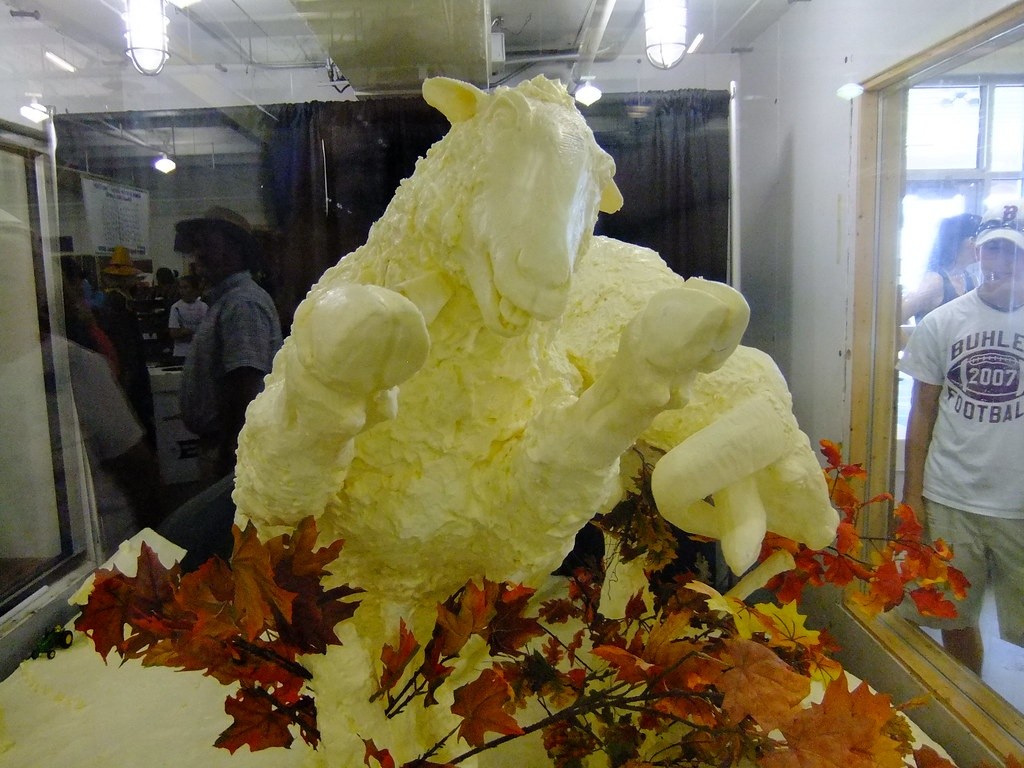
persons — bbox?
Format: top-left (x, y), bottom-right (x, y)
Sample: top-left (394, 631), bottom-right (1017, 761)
top-left (0, 232), bottom-right (296, 562)
top-left (899, 214), bottom-right (982, 331)
top-left (175, 206), bottom-right (284, 480)
top-left (900, 204), bottom-right (1024, 679)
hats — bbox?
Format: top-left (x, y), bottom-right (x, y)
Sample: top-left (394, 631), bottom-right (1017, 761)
top-left (972, 197), bottom-right (1024, 252)
top-left (171, 202), bottom-right (258, 257)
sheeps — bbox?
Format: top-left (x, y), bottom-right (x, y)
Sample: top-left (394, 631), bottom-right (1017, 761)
top-left (228, 73), bottom-right (838, 612)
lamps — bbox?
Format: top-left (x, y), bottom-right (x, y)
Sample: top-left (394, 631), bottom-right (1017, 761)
top-left (122, 0), bottom-right (171, 76)
top-left (644, 0), bottom-right (688, 70)
